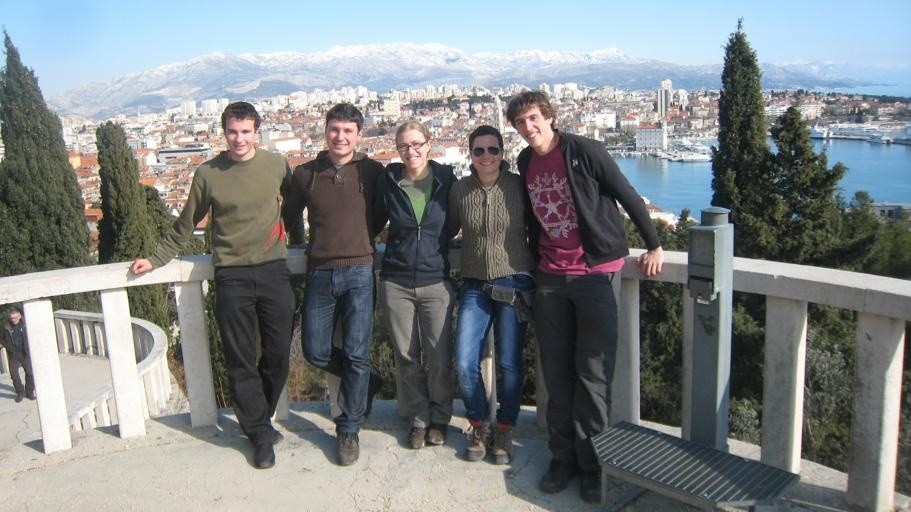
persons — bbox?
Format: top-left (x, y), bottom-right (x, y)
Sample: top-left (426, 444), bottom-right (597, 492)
top-left (506, 92), bottom-right (662, 503)
top-left (281, 104), bottom-right (390, 466)
top-left (445, 125), bottom-right (538, 466)
top-left (374, 121), bottom-right (456, 449)
top-left (131, 104), bottom-right (305, 470)
top-left (0, 309), bottom-right (36, 403)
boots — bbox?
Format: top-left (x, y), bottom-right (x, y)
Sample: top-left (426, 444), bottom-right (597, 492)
top-left (491, 421), bottom-right (513, 464)
top-left (464, 419), bottom-right (493, 461)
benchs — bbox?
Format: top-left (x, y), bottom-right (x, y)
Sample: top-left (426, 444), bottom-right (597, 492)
top-left (591, 421), bottom-right (800, 512)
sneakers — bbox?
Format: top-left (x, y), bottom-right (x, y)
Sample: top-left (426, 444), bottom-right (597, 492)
top-left (337, 432), bottom-right (359, 465)
top-left (254, 443), bottom-right (275, 469)
top-left (427, 424), bottom-right (449, 444)
top-left (408, 428), bottom-right (427, 448)
top-left (270, 426), bottom-right (283, 444)
top-left (581, 466), bottom-right (602, 501)
top-left (539, 453), bottom-right (577, 493)
top-left (364, 374), bottom-right (384, 417)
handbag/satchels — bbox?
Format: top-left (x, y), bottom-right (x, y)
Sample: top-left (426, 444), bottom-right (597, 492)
top-left (489, 285), bottom-right (534, 323)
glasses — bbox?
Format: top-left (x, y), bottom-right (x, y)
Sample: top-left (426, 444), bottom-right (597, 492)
top-left (398, 141), bottom-right (427, 152)
top-left (469, 147), bottom-right (502, 157)
